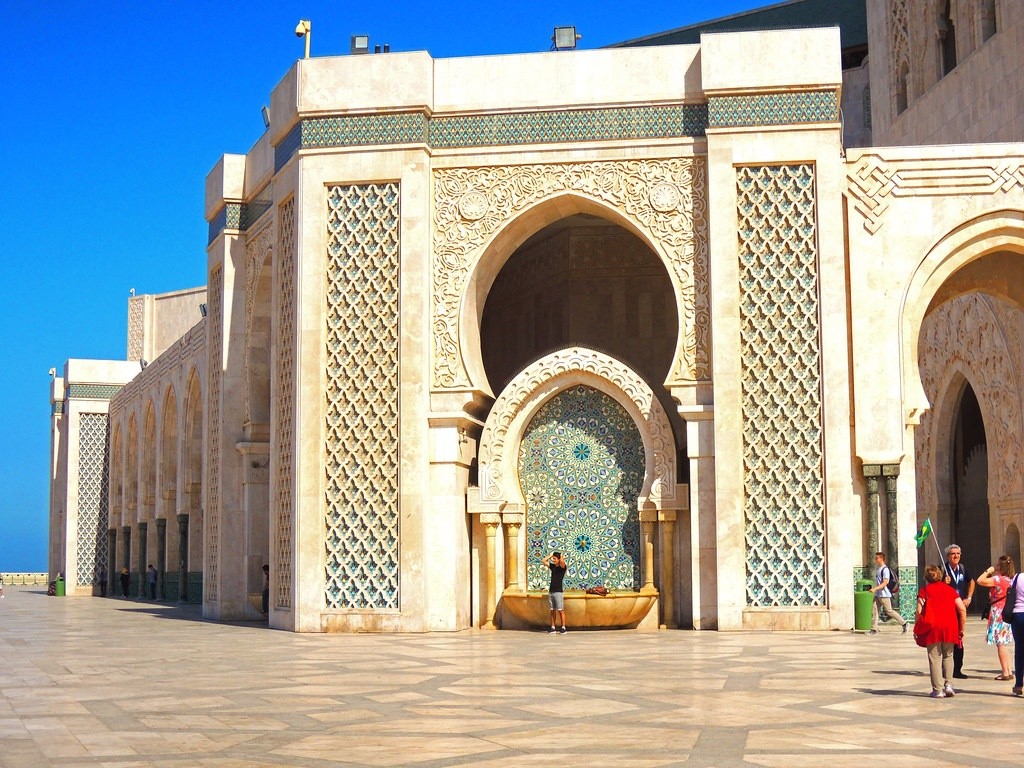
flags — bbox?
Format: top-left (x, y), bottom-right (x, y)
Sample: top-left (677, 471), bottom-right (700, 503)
top-left (914, 519), bottom-right (933, 548)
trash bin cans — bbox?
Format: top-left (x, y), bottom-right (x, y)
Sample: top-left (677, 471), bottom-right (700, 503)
top-left (55, 577), bottom-right (65, 596)
top-left (854, 579), bottom-right (874, 630)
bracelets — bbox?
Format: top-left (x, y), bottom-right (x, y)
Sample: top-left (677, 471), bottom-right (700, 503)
top-left (986, 570), bottom-right (991, 576)
top-left (968, 596), bottom-right (971, 601)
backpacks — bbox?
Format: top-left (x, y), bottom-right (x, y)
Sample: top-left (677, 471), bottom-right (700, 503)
top-left (881, 566), bottom-right (900, 593)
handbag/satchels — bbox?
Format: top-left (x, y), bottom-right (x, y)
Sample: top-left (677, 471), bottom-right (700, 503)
top-left (1002, 573), bottom-right (1021, 624)
top-left (981, 599), bottom-right (991, 620)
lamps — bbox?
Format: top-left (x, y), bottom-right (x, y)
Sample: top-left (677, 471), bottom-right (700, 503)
top-left (552, 26), bottom-right (580, 52)
top-left (199, 303), bottom-right (206, 317)
top-left (350, 35), bottom-right (368, 55)
top-left (140, 359), bottom-right (147, 369)
top-left (263, 107), bottom-right (271, 127)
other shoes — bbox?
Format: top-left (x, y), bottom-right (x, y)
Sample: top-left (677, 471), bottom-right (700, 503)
top-left (995, 675), bottom-right (1009, 680)
top-left (998, 675), bottom-right (1013, 679)
top-left (1013, 687), bottom-right (1023, 695)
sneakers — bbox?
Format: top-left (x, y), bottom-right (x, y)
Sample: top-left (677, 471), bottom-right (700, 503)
top-left (953, 673), bottom-right (967, 679)
top-left (865, 629), bottom-right (878, 635)
top-left (901, 622), bottom-right (910, 634)
top-left (547, 628), bottom-right (556, 634)
top-left (930, 690), bottom-right (946, 697)
top-left (944, 686), bottom-right (956, 696)
top-left (560, 628), bottom-right (567, 634)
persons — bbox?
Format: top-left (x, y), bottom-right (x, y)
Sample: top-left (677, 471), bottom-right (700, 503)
top-left (542, 552), bottom-right (567, 635)
top-left (99, 565), bottom-right (107, 597)
top-left (263, 564), bottom-right (269, 613)
top-left (1009, 573), bottom-right (1024, 695)
top-left (120, 568), bottom-right (129, 599)
top-left (0, 578), bottom-right (4, 598)
top-left (977, 555), bottom-right (1015, 680)
top-left (866, 552), bottom-right (910, 635)
top-left (913, 564), bottom-right (966, 697)
top-left (148, 565), bottom-right (156, 599)
top-left (938, 545), bottom-right (975, 679)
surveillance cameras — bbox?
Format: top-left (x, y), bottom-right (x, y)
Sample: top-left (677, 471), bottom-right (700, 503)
top-left (48, 370), bottom-right (53, 376)
top-left (296, 24), bottom-right (305, 38)
